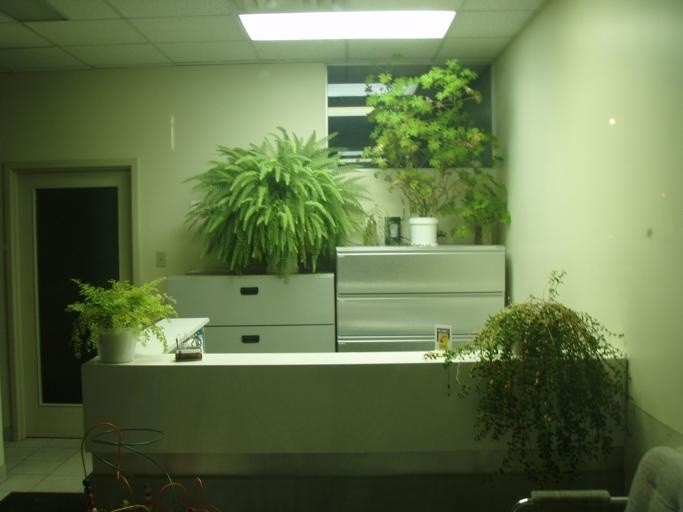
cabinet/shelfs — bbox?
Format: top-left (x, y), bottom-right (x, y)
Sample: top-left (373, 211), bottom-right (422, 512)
top-left (183, 267), bottom-right (335, 353)
top-left (335, 245), bottom-right (506, 352)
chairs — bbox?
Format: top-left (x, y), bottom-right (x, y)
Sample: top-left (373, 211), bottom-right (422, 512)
top-left (510, 446), bottom-right (682, 511)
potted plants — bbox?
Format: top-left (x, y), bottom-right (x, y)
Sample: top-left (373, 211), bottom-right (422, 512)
top-left (63, 276), bottom-right (180, 364)
top-left (359, 53), bottom-right (510, 245)
top-left (423, 269), bottom-right (636, 493)
top-left (178, 125), bottom-right (375, 276)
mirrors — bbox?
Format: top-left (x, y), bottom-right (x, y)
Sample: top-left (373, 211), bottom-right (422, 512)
top-left (324, 62), bottom-right (494, 173)
